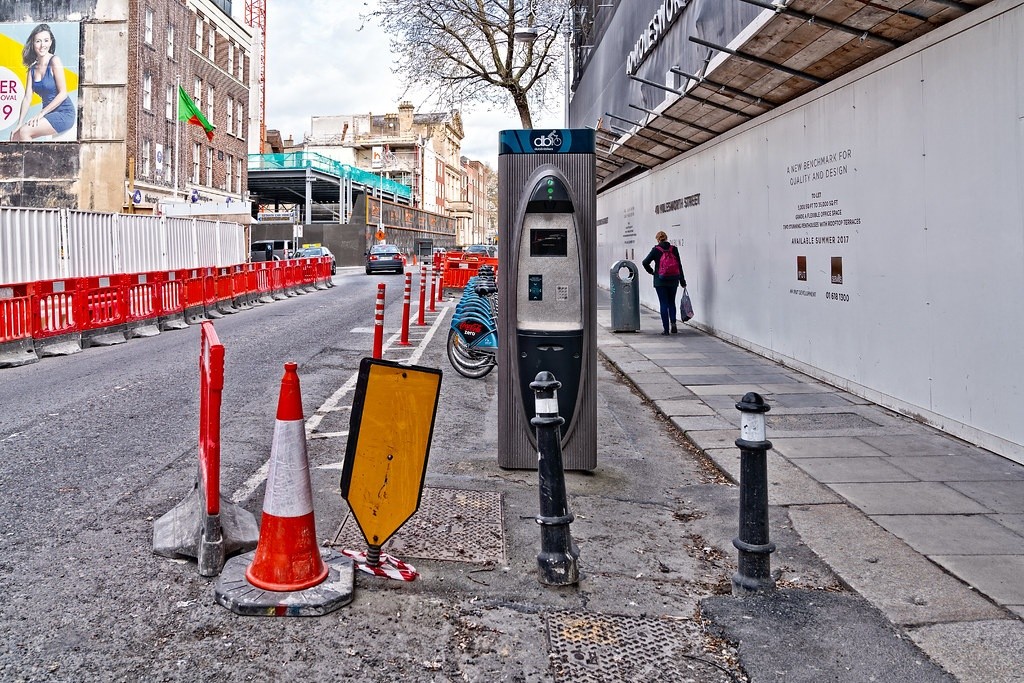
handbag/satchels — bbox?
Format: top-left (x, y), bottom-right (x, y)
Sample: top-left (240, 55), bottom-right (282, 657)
top-left (679, 287), bottom-right (694, 322)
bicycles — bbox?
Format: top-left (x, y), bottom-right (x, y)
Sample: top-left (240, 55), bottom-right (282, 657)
top-left (446, 265), bottom-right (500, 380)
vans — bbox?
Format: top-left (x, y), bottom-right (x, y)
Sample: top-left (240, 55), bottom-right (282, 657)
top-left (250, 239), bottom-right (297, 263)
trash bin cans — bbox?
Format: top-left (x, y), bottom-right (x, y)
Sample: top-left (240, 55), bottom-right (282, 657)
top-left (610, 260), bottom-right (640, 334)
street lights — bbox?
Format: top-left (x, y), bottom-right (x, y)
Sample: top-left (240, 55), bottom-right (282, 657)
top-left (512, 4), bottom-right (575, 129)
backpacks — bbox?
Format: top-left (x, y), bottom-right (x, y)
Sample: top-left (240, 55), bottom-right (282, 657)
top-left (656, 245), bottom-right (680, 276)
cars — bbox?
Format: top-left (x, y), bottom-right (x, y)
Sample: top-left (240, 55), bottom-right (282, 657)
top-left (364, 245), bottom-right (404, 274)
top-left (421, 248), bottom-right (447, 265)
top-left (292, 243), bottom-right (336, 275)
top-left (462, 244), bottom-right (496, 257)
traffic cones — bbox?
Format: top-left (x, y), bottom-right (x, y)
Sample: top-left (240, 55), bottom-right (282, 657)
top-left (402, 253), bottom-right (407, 266)
top-left (411, 255), bottom-right (419, 266)
top-left (214, 362), bottom-right (356, 617)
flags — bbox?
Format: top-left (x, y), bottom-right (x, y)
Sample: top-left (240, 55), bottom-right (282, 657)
top-left (179, 84), bottom-right (215, 141)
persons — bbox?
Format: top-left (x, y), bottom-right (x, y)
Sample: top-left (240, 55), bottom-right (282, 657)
top-left (14, 25), bottom-right (75, 140)
top-left (643, 233), bottom-right (686, 335)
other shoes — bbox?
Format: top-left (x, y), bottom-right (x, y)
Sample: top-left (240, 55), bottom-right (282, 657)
top-left (671, 326), bottom-right (677, 333)
top-left (661, 331), bottom-right (669, 335)
